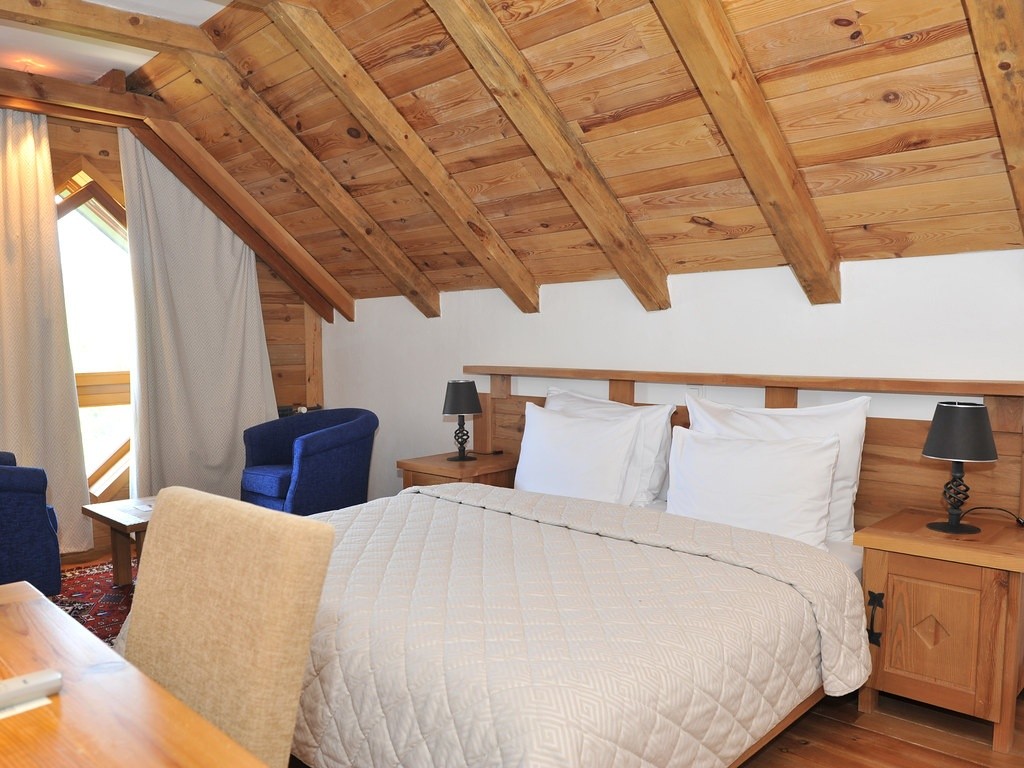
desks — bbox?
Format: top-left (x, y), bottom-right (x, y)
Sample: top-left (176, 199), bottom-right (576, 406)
top-left (0, 579), bottom-right (274, 768)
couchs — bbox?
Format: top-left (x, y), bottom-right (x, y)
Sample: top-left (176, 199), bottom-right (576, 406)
top-left (0, 450), bottom-right (63, 597)
top-left (240, 408), bottom-right (379, 517)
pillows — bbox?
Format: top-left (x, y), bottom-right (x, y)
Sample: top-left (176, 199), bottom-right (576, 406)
top-left (664, 426), bottom-right (841, 553)
top-left (544, 386), bottom-right (676, 508)
top-left (684, 391), bottom-right (870, 542)
top-left (513, 402), bottom-right (643, 504)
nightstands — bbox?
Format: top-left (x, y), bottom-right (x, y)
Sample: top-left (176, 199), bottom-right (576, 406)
top-left (396, 449), bottom-right (518, 489)
top-left (851, 508), bottom-right (1024, 756)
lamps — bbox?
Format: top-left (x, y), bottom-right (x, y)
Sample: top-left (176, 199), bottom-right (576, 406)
top-left (919, 400), bottom-right (999, 534)
top-left (442, 379), bottom-right (483, 462)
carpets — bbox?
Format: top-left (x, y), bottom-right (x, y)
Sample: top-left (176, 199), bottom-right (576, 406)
top-left (46, 558), bottom-right (138, 648)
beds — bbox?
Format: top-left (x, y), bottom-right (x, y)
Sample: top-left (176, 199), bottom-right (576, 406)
top-left (222, 365), bottom-right (1024, 768)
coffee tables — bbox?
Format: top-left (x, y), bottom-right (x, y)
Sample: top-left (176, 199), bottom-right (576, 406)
top-left (83, 496), bottom-right (156, 587)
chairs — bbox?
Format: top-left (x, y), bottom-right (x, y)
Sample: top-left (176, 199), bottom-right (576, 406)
top-left (111, 485), bottom-right (334, 768)
